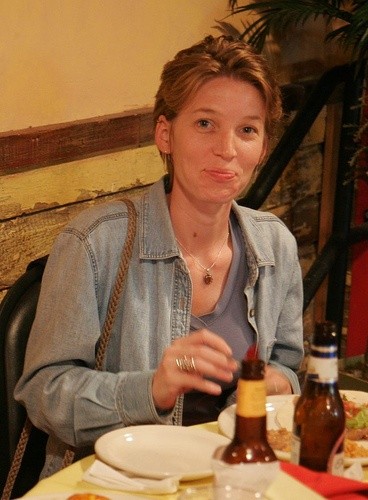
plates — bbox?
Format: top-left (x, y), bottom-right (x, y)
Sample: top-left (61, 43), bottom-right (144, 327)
top-left (217, 394), bottom-right (368, 465)
top-left (277, 389), bottom-right (367, 463)
top-left (94, 424), bottom-right (232, 481)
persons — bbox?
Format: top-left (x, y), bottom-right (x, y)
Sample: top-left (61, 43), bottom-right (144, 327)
top-left (13, 34), bottom-right (305, 483)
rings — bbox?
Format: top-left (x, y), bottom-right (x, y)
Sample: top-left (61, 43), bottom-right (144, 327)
top-left (175, 354), bottom-right (201, 376)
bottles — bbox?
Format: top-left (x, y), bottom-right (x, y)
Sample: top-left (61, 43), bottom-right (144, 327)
top-left (221, 344), bottom-right (278, 465)
top-left (293, 319), bottom-right (347, 472)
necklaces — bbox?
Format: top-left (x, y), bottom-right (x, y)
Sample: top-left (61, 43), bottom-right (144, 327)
top-left (173, 232), bottom-right (229, 286)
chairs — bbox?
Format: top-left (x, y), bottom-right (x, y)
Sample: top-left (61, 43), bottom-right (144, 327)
top-left (1, 265), bottom-right (48, 500)
